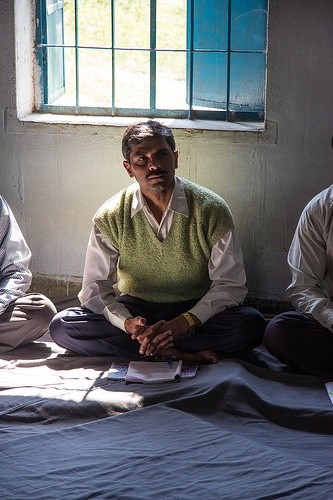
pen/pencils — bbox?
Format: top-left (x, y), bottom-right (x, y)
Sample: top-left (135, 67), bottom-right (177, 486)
top-left (167, 358), bottom-right (171, 368)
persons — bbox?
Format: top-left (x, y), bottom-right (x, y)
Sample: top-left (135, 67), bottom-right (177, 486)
top-left (263, 135), bottom-right (333, 380)
top-left (0, 194), bottom-right (57, 356)
top-left (48, 120), bottom-right (266, 366)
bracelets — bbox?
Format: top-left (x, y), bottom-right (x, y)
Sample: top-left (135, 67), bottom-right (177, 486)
top-left (180, 313), bottom-right (195, 338)
